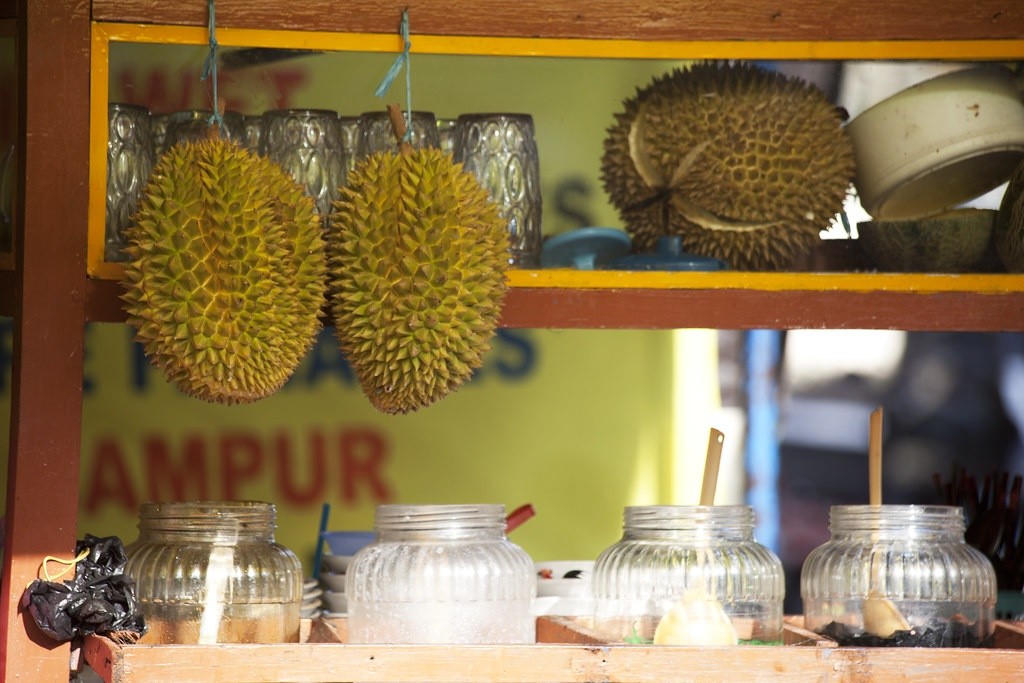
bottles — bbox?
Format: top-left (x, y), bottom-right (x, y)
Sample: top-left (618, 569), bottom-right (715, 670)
top-left (587, 505), bottom-right (787, 648)
top-left (103, 101), bottom-right (541, 281)
top-left (118, 498), bottom-right (304, 645)
top-left (346, 504), bottom-right (540, 646)
top-left (800, 504), bottom-right (999, 650)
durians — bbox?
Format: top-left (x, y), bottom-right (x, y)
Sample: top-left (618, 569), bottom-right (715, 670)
top-left (121, 136), bottom-right (332, 406)
top-left (602, 58), bottom-right (861, 273)
top-left (327, 103), bottom-right (520, 413)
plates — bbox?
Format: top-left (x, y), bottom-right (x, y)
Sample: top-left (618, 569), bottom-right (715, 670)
top-left (295, 529), bottom-right (380, 620)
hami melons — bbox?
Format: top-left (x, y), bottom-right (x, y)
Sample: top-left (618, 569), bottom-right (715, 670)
top-left (857, 208), bottom-right (998, 274)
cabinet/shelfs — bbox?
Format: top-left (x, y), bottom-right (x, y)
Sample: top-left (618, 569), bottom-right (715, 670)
top-left (0, 1), bottom-right (1024, 683)
